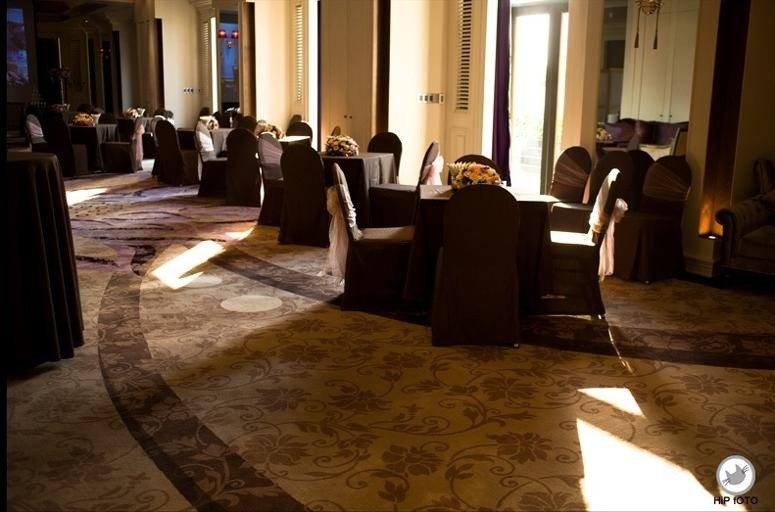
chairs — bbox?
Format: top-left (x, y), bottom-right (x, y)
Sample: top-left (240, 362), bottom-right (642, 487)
top-left (327, 161), bottom-right (428, 310)
top-left (540, 165), bottom-right (623, 339)
top-left (368, 130), bottom-right (402, 178)
top-left (432, 187), bottom-right (522, 346)
top-left (446, 155), bottom-right (503, 186)
top-left (554, 118), bottom-right (695, 283)
top-left (714, 156), bottom-right (775, 285)
top-left (371, 140), bottom-right (444, 228)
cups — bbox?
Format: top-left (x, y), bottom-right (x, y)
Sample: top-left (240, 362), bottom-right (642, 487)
top-left (502, 181), bottom-right (507, 188)
top-left (608, 114), bottom-right (616, 123)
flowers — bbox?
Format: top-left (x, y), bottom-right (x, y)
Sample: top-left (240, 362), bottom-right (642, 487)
top-left (452, 163), bottom-right (501, 187)
top-left (324, 134), bottom-right (360, 158)
